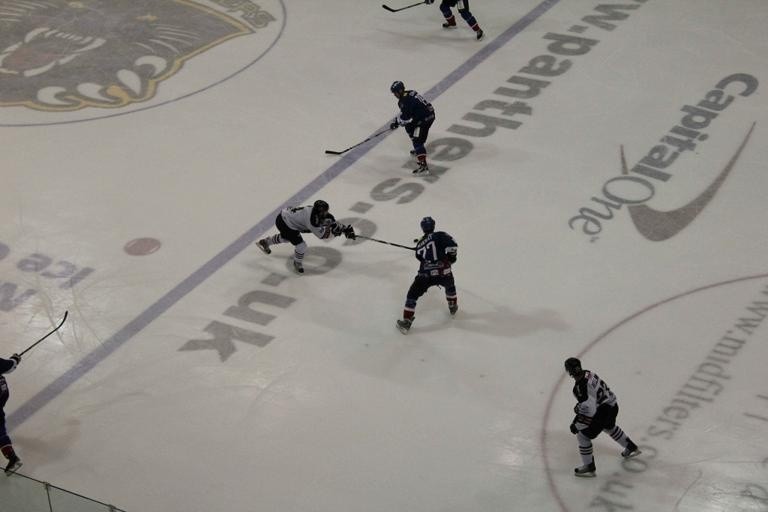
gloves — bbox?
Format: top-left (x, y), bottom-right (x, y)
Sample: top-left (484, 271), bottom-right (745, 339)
top-left (10, 353), bottom-right (22, 365)
top-left (331, 223), bottom-right (342, 237)
top-left (570, 423), bottom-right (579, 434)
top-left (390, 117), bottom-right (400, 129)
top-left (425, 0), bottom-right (434, 4)
top-left (345, 225), bottom-right (355, 240)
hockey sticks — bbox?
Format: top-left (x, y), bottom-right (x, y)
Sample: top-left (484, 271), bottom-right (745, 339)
top-left (326, 126), bottom-right (398, 155)
top-left (19, 310), bottom-right (68, 359)
top-left (382, 2), bottom-right (424, 12)
top-left (354, 234), bottom-right (417, 251)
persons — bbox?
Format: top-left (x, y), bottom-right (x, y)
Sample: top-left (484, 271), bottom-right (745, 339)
top-left (0, 353), bottom-right (22, 473)
top-left (397, 217), bottom-right (458, 331)
top-left (423, 0), bottom-right (483, 40)
top-left (390, 81), bottom-right (435, 174)
top-left (260, 200), bottom-right (355, 273)
top-left (565, 357), bottom-right (637, 473)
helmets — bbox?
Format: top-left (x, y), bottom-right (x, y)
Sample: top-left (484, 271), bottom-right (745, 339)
top-left (391, 81), bottom-right (405, 92)
top-left (565, 358), bottom-right (582, 375)
top-left (314, 200), bottom-right (329, 212)
top-left (420, 217), bottom-right (435, 231)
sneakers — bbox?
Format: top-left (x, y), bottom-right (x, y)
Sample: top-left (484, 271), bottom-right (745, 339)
top-left (443, 15), bottom-right (456, 27)
top-left (477, 28), bottom-right (483, 39)
top-left (410, 150), bottom-right (416, 155)
top-left (449, 305), bottom-right (458, 314)
top-left (621, 437), bottom-right (637, 456)
top-left (5, 451), bottom-right (19, 472)
top-left (575, 455), bottom-right (596, 473)
top-left (260, 239), bottom-right (271, 254)
top-left (413, 160), bottom-right (428, 174)
top-left (397, 317), bottom-right (415, 330)
top-left (294, 260), bottom-right (303, 273)
